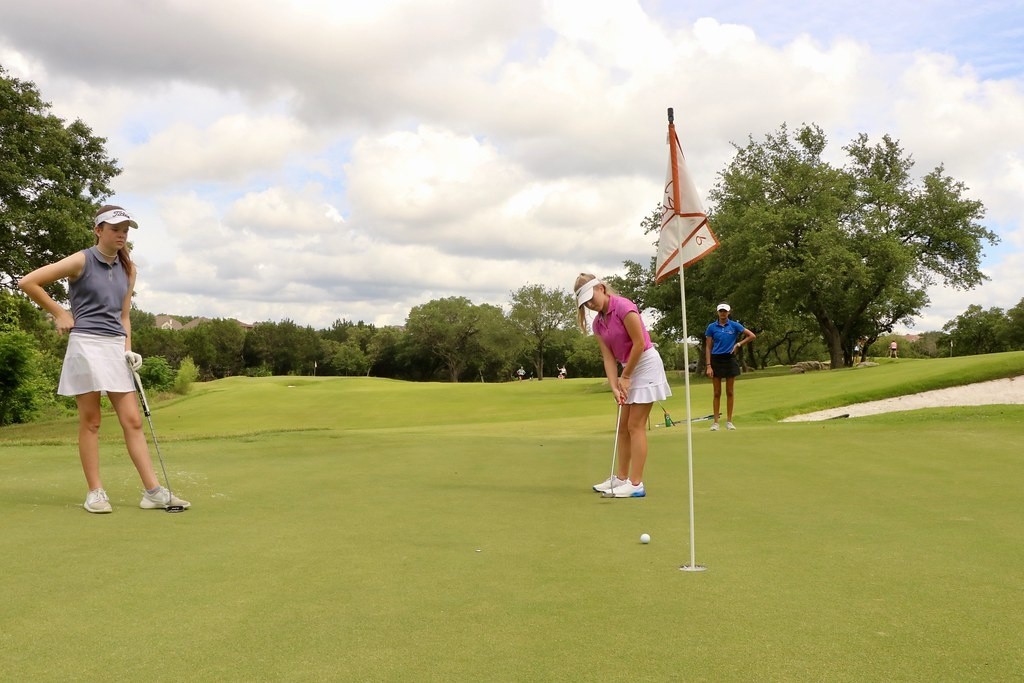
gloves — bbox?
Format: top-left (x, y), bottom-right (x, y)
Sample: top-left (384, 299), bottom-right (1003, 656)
top-left (125, 351), bottom-right (142, 371)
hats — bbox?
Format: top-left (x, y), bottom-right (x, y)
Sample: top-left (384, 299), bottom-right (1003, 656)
top-left (575, 277), bottom-right (602, 310)
top-left (717, 304), bottom-right (730, 312)
top-left (94, 208), bottom-right (138, 230)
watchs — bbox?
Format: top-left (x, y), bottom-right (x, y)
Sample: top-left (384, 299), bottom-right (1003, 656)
top-left (737, 343), bottom-right (740, 347)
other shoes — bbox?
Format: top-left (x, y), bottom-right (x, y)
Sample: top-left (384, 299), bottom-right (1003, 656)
top-left (710, 424), bottom-right (719, 431)
top-left (727, 422), bottom-right (736, 430)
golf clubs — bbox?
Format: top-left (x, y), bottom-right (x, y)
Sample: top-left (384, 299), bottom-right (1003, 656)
top-left (603, 396), bottom-right (624, 498)
top-left (129, 360), bottom-right (185, 513)
top-left (658, 401), bottom-right (676, 426)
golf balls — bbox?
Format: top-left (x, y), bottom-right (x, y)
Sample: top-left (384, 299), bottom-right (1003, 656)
top-left (640, 533), bottom-right (651, 544)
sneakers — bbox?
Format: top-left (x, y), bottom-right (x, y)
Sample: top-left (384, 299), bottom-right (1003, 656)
top-left (84, 488), bottom-right (113, 513)
top-left (592, 475), bottom-right (646, 497)
top-left (140, 486), bottom-right (191, 509)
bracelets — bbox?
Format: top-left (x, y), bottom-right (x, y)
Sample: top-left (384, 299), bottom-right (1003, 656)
top-left (706, 364), bottom-right (711, 365)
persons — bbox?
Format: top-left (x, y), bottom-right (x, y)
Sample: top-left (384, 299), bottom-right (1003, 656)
top-left (17, 205), bottom-right (190, 514)
top-left (517, 366), bottom-right (526, 380)
top-left (705, 302), bottom-right (757, 432)
top-left (558, 372), bottom-right (565, 379)
top-left (557, 364), bottom-right (567, 377)
top-left (853, 344), bottom-right (859, 363)
top-left (574, 273), bottom-right (673, 498)
top-left (888, 340), bottom-right (898, 359)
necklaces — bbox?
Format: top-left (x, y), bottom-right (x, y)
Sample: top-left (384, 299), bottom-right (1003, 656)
top-left (98, 249), bottom-right (117, 258)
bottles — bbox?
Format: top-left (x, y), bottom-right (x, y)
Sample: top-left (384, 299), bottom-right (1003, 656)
top-left (664, 411), bottom-right (670, 427)
